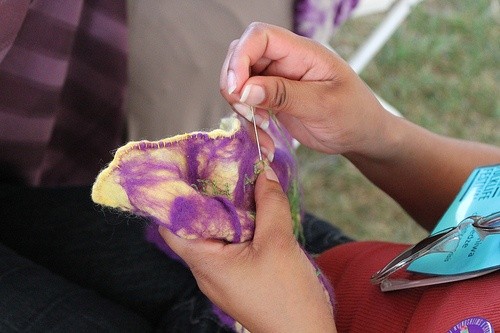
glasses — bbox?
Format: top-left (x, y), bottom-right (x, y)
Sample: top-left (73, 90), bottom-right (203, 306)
top-left (370, 216), bottom-right (500, 292)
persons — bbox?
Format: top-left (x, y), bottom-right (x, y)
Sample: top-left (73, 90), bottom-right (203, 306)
top-left (0, 22), bottom-right (500, 333)
